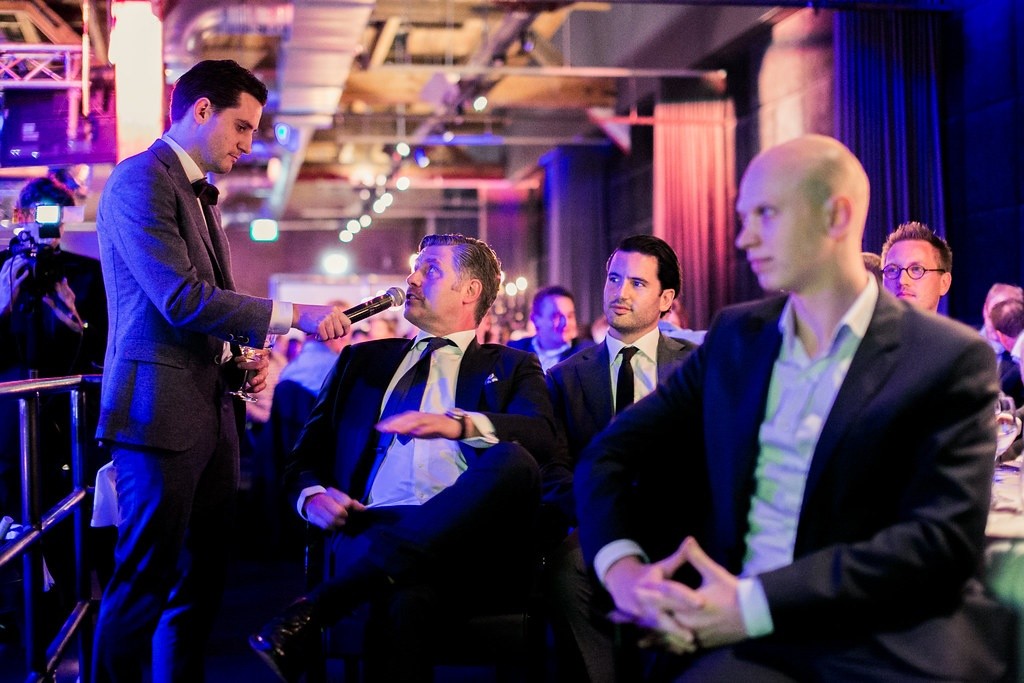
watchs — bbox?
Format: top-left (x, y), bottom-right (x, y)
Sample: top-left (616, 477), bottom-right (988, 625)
top-left (445, 408), bottom-right (466, 440)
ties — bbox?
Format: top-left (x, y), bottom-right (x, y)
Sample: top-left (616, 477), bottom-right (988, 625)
top-left (615, 344), bottom-right (639, 416)
top-left (355, 332), bottom-right (455, 505)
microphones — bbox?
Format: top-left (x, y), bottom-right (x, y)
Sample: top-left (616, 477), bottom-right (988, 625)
top-left (342, 287), bottom-right (406, 324)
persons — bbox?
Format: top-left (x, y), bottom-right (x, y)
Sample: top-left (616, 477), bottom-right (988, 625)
top-left (250, 234), bottom-right (560, 683)
top-left (279, 300), bottom-right (352, 394)
top-left (0, 178), bottom-right (109, 646)
top-left (96, 59), bottom-right (351, 683)
top-left (537, 234), bottom-right (700, 683)
top-left (572, 134), bottom-right (1017, 683)
top-left (880, 221), bottom-right (953, 312)
top-left (275, 333), bottom-right (302, 362)
top-left (977, 284), bottom-right (1024, 409)
top-left (507, 286), bottom-right (590, 375)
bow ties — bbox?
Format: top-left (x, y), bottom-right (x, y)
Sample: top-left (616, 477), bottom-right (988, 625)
top-left (194, 178), bottom-right (220, 208)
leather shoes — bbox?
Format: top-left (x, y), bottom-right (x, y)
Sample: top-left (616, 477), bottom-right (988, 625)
top-left (248, 597), bottom-right (326, 683)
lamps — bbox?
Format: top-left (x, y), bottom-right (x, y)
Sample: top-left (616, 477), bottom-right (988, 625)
top-left (436, 117), bottom-right (459, 143)
top-left (518, 28), bottom-right (536, 56)
top-left (411, 148), bottom-right (431, 168)
top-left (468, 84), bottom-right (487, 112)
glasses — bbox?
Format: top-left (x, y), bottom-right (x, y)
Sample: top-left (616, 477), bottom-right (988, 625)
top-left (880, 263), bottom-right (947, 280)
